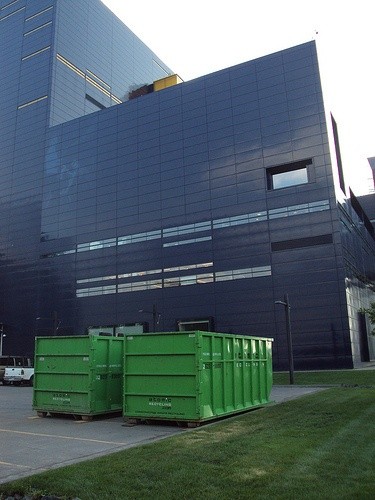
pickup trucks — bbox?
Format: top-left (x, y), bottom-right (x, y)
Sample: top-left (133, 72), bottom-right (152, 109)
top-left (2, 366), bottom-right (34, 386)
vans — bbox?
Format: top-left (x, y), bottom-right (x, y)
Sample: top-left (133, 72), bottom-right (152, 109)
top-left (1, 355), bottom-right (32, 384)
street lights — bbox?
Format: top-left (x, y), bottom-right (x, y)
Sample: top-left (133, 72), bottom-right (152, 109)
top-left (36, 315), bottom-right (62, 335)
top-left (276, 293), bottom-right (295, 386)
top-left (139, 304), bottom-right (157, 332)
